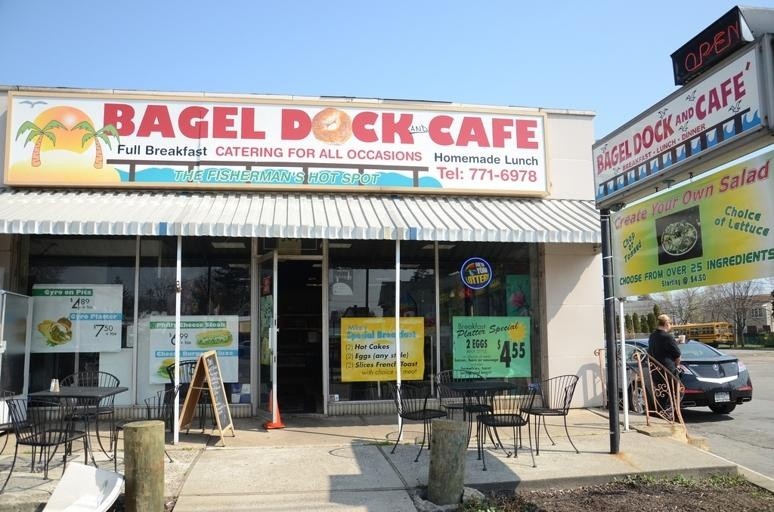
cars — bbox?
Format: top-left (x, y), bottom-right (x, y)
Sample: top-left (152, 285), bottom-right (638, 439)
top-left (605, 336), bottom-right (752, 417)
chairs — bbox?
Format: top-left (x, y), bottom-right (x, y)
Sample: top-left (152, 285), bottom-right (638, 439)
top-left (385, 366), bottom-right (583, 469)
top-left (0, 359), bottom-right (219, 493)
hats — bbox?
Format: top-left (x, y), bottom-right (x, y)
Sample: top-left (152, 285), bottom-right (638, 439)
top-left (658, 314), bottom-right (671, 323)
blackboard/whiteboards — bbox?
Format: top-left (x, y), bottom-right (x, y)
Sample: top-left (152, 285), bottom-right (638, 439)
top-left (203, 350), bottom-right (232, 435)
top-left (179, 352), bottom-right (205, 431)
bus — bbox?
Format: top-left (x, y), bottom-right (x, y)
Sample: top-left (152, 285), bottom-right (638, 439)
top-left (668, 321), bottom-right (735, 348)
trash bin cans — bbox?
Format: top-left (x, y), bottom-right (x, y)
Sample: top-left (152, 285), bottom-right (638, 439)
top-left (329, 368), bottom-right (352, 401)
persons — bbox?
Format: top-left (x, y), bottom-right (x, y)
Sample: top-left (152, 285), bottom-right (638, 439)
top-left (648, 314), bottom-right (685, 420)
